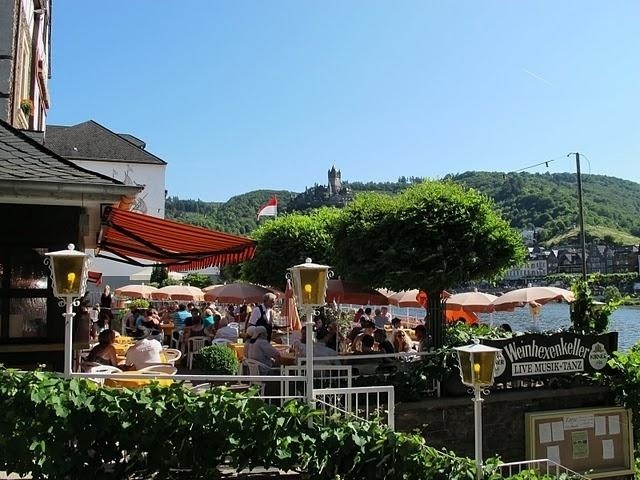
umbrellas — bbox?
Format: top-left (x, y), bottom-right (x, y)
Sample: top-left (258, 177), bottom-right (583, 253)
top-left (201, 279), bottom-right (227, 292)
top-left (325, 280), bottom-right (389, 354)
top-left (387, 289), bottom-right (420, 329)
top-left (489, 283), bottom-right (575, 332)
top-left (150, 282), bottom-right (205, 305)
top-left (114, 283), bottom-right (158, 299)
top-left (439, 291), bottom-right (499, 313)
top-left (416, 289), bottom-right (479, 324)
top-left (281, 279), bottom-right (304, 344)
top-left (203, 281), bottom-right (274, 322)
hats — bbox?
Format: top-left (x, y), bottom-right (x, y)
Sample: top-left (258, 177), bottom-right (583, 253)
top-left (246, 325), bottom-right (268, 341)
top-left (133, 325), bottom-right (151, 340)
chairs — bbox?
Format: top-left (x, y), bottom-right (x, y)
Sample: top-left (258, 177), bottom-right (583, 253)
top-left (282, 303), bottom-right (437, 395)
top-left (81, 300), bottom-right (281, 398)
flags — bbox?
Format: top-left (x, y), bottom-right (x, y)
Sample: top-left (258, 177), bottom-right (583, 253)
top-left (256, 198), bottom-right (278, 216)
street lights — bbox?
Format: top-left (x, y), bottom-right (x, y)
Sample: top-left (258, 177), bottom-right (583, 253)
top-left (453, 338), bottom-right (503, 480)
top-left (42, 242), bottom-right (96, 383)
top-left (285, 255), bottom-right (334, 429)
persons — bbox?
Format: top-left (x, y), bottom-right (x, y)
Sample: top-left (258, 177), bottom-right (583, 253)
top-left (246, 292), bottom-right (277, 344)
top-left (18, 265), bottom-right (48, 339)
top-left (97, 284), bottom-right (119, 330)
